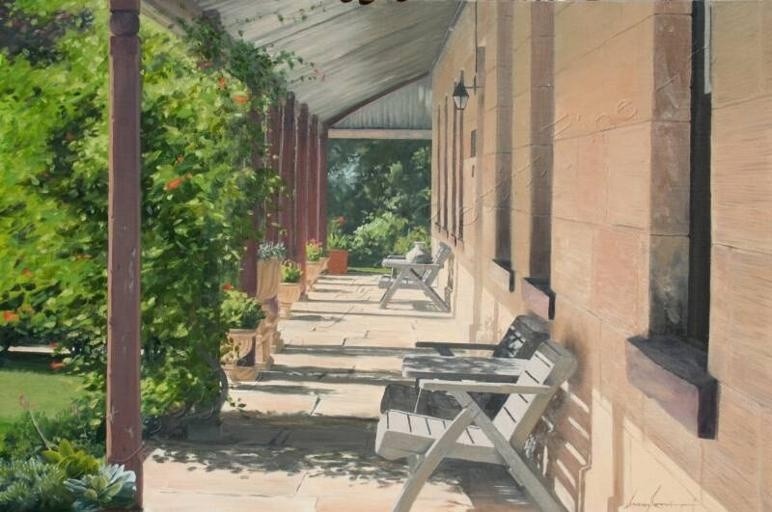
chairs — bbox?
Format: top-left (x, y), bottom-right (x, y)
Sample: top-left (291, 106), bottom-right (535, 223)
top-left (375, 340), bottom-right (576, 512)
top-left (378, 242), bottom-right (450, 312)
top-left (380, 316), bottom-right (550, 426)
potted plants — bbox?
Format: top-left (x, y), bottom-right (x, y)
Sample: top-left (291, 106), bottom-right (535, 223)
top-left (319, 246), bottom-right (330, 270)
top-left (327, 233), bottom-right (348, 274)
top-left (277, 260), bottom-right (304, 318)
top-left (219, 290), bottom-right (267, 389)
top-left (305, 238), bottom-right (323, 291)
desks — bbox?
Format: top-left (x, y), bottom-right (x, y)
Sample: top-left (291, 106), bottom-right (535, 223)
top-left (401, 354), bottom-right (529, 415)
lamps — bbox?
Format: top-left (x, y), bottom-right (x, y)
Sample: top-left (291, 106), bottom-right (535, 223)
top-left (453, 76), bottom-right (476, 111)
